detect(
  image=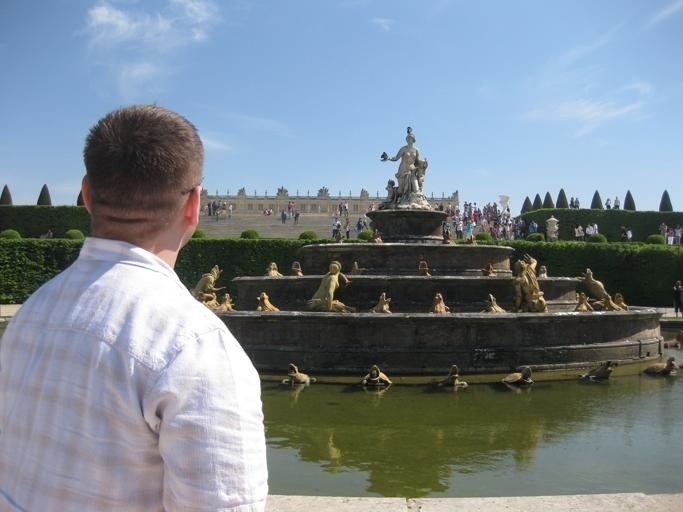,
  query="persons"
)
[658,223,682,245]
[281,200,301,226]
[201,198,234,222]
[618,224,631,242]
[262,206,274,217]
[574,220,599,241]
[605,197,612,210]
[431,201,538,242]
[330,198,375,239]
[0,103,270,512]
[378,126,435,212]
[613,197,620,209]
[570,196,580,209]
[671,278,683,318]
